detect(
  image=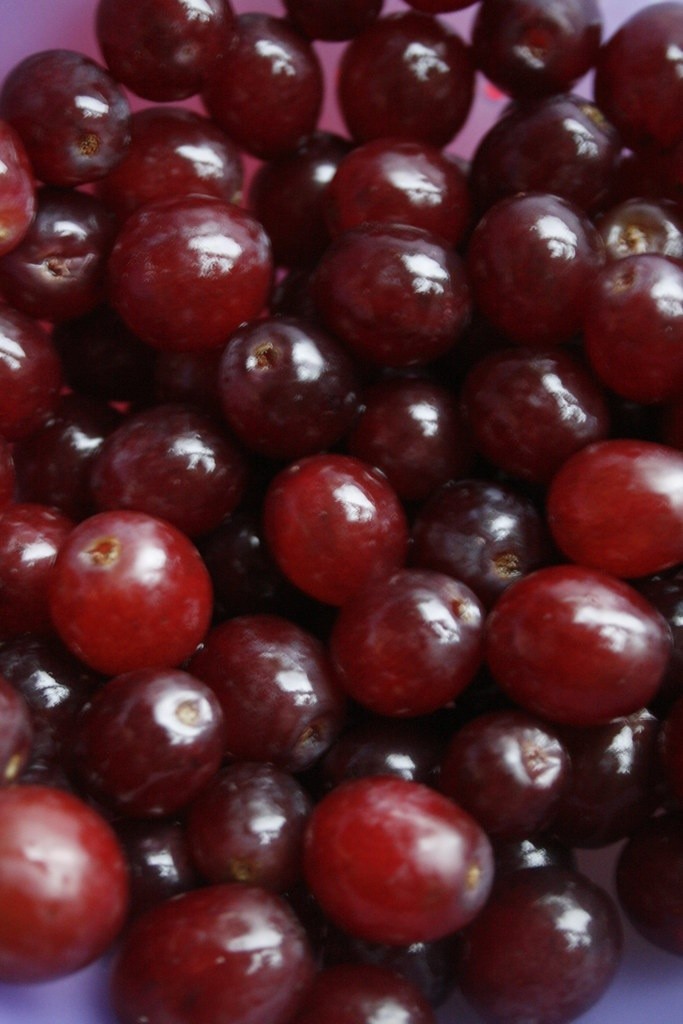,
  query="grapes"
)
[0,0,683,1024]
